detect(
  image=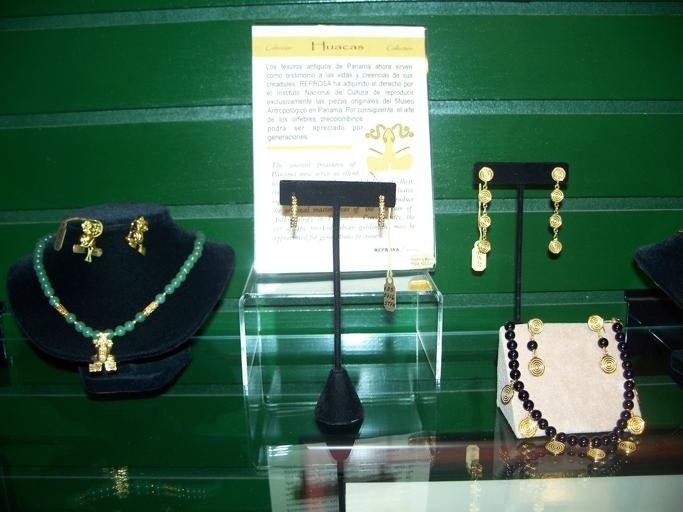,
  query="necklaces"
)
[32,227,206,373]
[498,317,645,463]
